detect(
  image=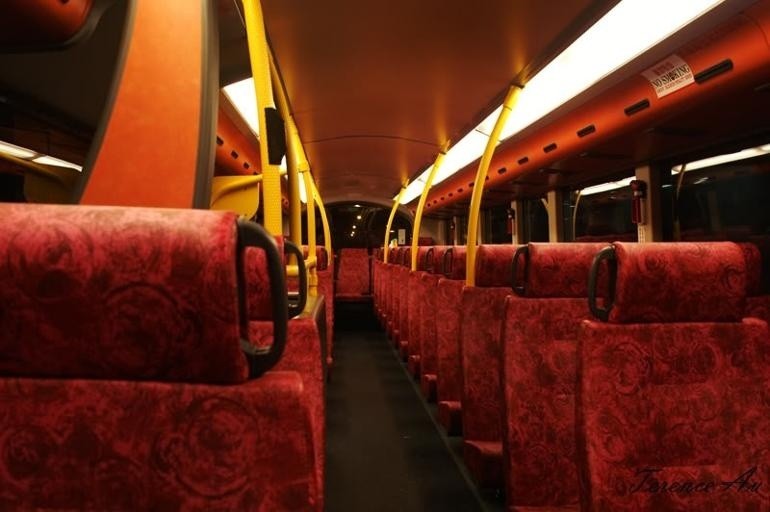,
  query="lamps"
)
[220,63,314,203]
